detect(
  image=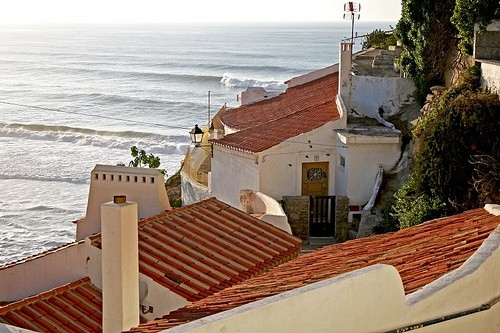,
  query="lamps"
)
[189,124,206,147]
